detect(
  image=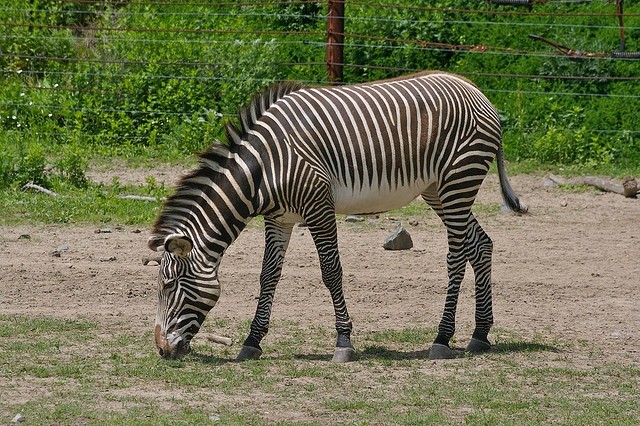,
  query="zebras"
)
[146,69,530,364]
